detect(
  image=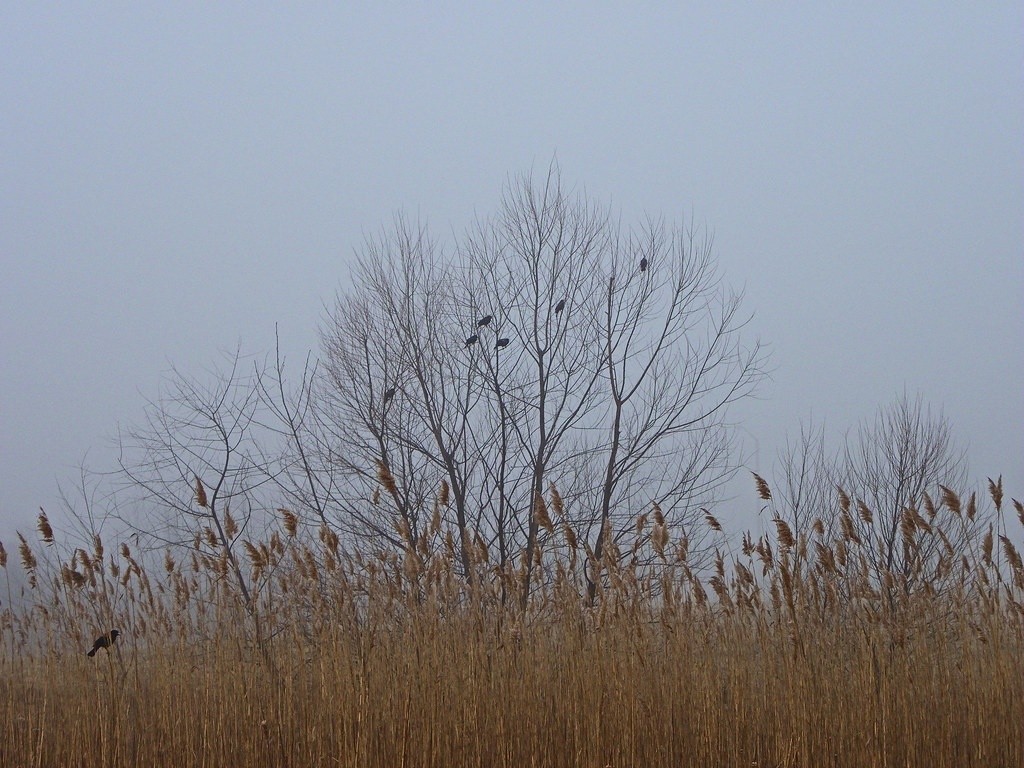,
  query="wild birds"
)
[475,315,492,330]
[87,629,121,658]
[462,335,478,350]
[554,299,566,315]
[640,257,647,271]
[493,338,507,349]
[385,389,394,402]
